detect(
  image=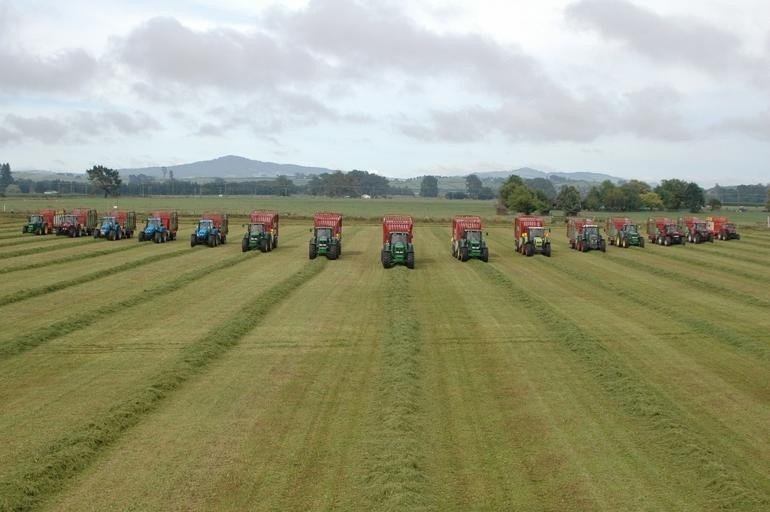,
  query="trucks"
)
[451,214,490,263]
[22,207,137,242]
[604,215,742,249]
[137,211,179,243]
[242,209,279,252]
[514,216,551,257]
[566,217,606,252]
[381,214,415,269]
[191,211,229,248]
[308,212,344,260]
